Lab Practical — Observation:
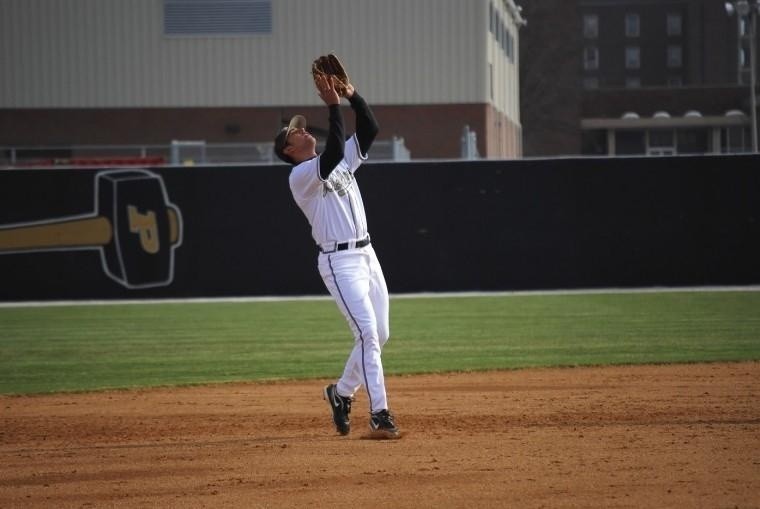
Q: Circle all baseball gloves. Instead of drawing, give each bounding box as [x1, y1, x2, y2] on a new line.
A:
[311, 54, 349, 98]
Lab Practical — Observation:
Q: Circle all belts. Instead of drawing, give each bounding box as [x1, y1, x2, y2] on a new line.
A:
[316, 234, 371, 252]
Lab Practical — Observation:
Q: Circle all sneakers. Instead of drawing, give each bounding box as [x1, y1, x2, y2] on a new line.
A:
[368, 409, 400, 438]
[324, 384, 352, 436]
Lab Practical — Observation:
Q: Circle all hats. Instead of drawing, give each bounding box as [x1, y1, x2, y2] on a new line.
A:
[274, 114, 307, 161]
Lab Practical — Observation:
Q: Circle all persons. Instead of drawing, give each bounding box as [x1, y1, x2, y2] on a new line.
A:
[274, 54, 406, 438]
